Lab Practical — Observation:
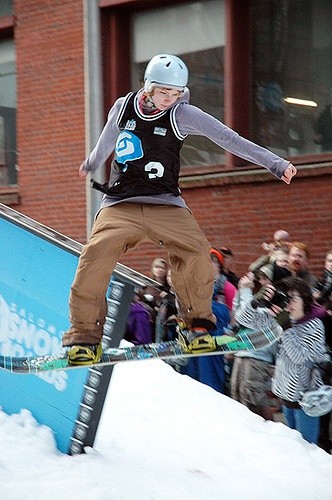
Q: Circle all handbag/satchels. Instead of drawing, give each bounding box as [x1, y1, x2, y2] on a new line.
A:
[297, 364, 332, 417]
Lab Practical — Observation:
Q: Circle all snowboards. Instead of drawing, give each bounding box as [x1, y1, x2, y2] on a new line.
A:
[0, 324, 282, 375]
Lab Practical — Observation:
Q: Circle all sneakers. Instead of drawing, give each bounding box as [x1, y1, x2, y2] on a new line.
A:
[67, 340, 102, 365]
[176, 322, 216, 353]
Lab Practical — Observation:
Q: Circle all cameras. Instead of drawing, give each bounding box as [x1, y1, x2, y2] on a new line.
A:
[267, 287, 290, 308]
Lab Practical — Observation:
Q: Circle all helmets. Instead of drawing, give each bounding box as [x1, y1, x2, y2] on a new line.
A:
[143, 54, 189, 95]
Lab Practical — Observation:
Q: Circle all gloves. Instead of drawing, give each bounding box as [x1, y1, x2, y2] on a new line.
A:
[253, 284, 266, 308]
[274, 311, 292, 331]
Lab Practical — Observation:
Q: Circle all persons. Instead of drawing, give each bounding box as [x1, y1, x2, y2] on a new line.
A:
[311, 251, 332, 316]
[61, 54, 297, 365]
[123, 301, 153, 346]
[160, 269, 178, 341]
[207, 247, 237, 309]
[234, 277, 331, 445]
[284, 242, 318, 289]
[181, 275, 230, 392]
[249, 229, 290, 274]
[139, 258, 169, 312]
[228, 263, 292, 422]
[219, 247, 240, 289]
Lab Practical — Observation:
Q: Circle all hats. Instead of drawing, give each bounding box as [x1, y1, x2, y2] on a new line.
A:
[219, 246, 233, 255]
[210, 246, 225, 265]
